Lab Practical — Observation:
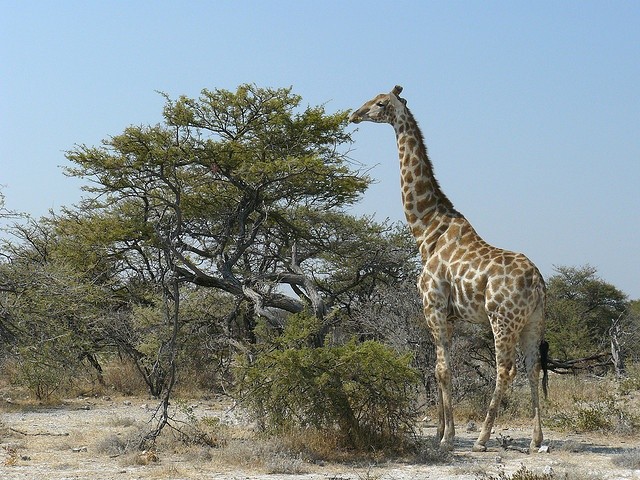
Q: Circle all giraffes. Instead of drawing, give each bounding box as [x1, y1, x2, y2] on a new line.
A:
[350, 85, 549, 453]
